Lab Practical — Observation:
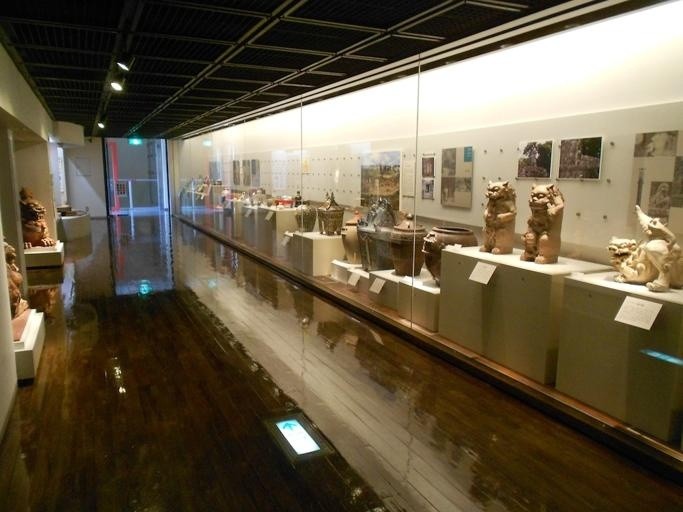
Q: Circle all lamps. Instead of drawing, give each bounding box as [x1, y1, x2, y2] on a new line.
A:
[110, 52, 135, 92]
[97, 114, 109, 129]
